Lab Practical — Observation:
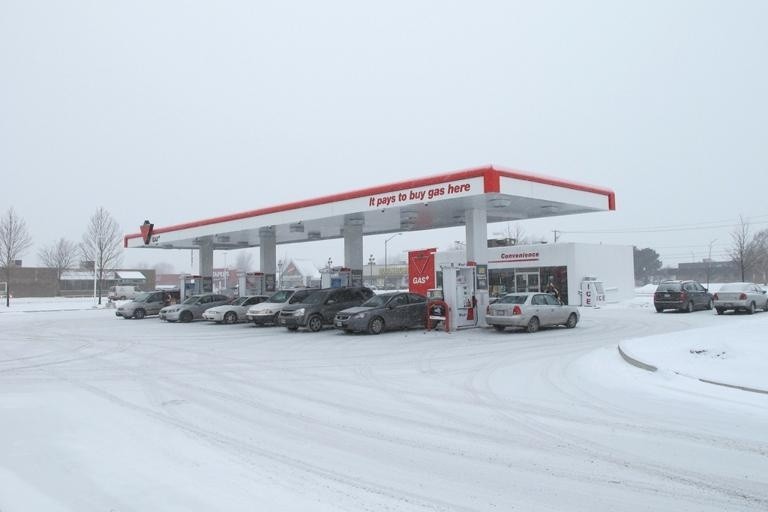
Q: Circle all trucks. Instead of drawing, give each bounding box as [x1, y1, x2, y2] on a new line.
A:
[107, 286, 135, 301]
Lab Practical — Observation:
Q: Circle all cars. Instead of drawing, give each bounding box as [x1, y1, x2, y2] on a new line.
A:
[485, 292, 580, 333]
[654, 280, 768, 314]
